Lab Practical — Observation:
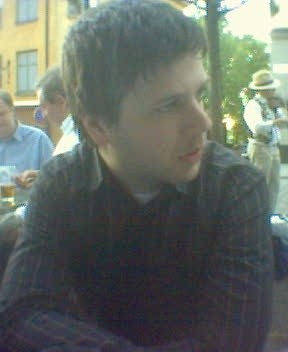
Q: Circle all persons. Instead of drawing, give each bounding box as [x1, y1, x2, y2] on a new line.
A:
[0, 1, 272, 351]
[36, 64, 80, 158]
[244, 69, 287, 228]
[1, 93, 53, 186]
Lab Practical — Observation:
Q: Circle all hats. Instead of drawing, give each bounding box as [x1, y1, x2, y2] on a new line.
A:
[247, 69, 281, 90]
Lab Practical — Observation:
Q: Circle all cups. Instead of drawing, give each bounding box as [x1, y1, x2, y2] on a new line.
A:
[0, 166, 17, 206]
